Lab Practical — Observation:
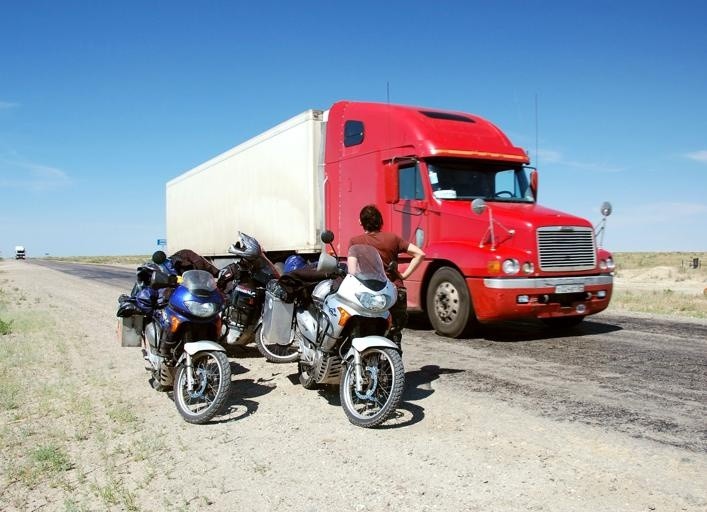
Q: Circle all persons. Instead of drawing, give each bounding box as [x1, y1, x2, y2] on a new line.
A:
[348, 205, 426, 347]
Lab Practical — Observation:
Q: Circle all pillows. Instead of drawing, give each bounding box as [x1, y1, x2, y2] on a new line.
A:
[227, 230, 262, 261]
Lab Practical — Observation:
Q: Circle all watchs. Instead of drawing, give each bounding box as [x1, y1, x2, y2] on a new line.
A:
[117, 249, 234, 420]
[286, 231, 409, 430]
[215, 235, 268, 347]
